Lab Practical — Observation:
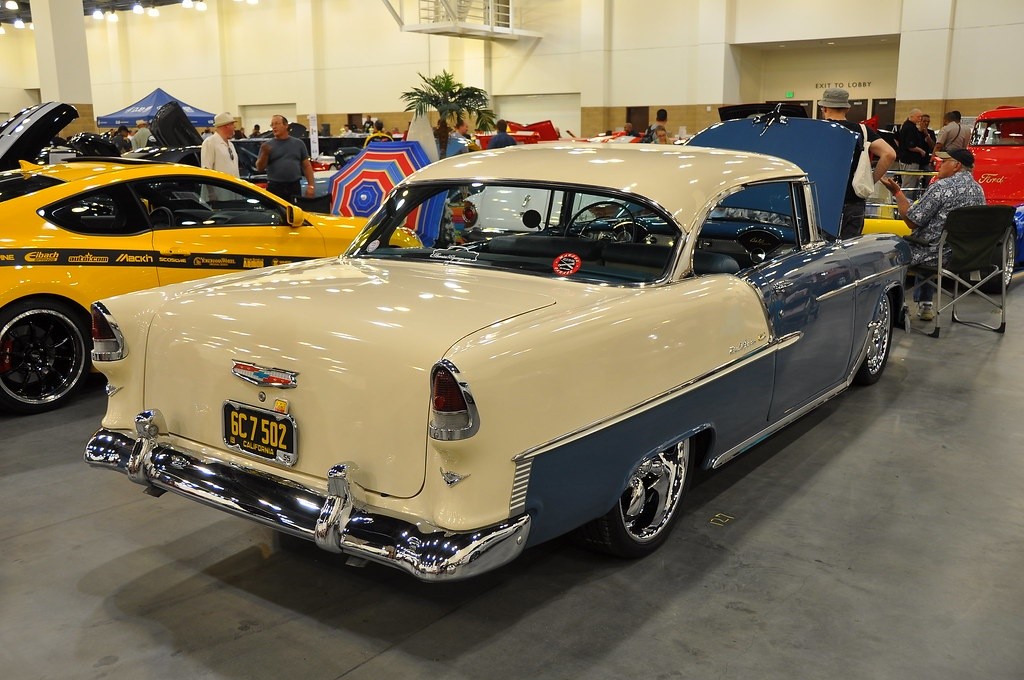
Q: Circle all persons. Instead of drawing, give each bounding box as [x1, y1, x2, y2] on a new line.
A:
[624, 123, 640, 138]
[256, 115, 314, 208]
[102, 120, 153, 153]
[363, 120, 394, 149]
[249, 124, 261, 138]
[880, 148, 986, 320]
[341, 115, 399, 133]
[642, 109, 673, 145]
[818, 87, 896, 239]
[487, 120, 517, 149]
[896, 108, 971, 198]
[604, 130, 612, 136]
[431, 118, 472, 158]
[401, 122, 411, 141]
[199, 112, 246, 210]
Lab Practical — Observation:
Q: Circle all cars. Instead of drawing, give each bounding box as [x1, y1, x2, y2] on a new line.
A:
[0, 158, 427, 414]
[461, 182, 610, 233]
[929, 106, 1024, 209]
[309, 135, 403, 173]
[0, 100, 80, 171]
[109, 103, 341, 213]
[869, 127, 922, 201]
[79, 103, 910, 584]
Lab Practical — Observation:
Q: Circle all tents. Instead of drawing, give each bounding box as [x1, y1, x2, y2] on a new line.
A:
[96, 87, 218, 136]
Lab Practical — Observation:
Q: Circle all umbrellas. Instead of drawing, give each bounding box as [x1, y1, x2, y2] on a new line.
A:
[327, 141, 449, 247]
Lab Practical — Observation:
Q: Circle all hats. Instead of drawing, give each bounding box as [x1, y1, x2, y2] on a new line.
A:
[213, 112, 238, 127]
[818, 88, 851, 108]
[136, 120, 148, 126]
[934, 147, 975, 168]
[119, 125, 130, 133]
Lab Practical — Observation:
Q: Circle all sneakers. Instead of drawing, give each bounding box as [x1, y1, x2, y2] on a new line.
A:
[917, 302, 932, 320]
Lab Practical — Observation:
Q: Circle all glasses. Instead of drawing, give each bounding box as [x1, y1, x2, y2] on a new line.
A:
[228, 148, 235, 161]
[270, 122, 285, 128]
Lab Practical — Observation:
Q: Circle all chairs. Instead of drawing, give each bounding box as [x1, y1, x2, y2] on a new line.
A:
[899, 205, 1017, 339]
[487, 235, 740, 277]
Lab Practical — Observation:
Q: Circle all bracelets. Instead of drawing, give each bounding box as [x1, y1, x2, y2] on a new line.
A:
[308, 185, 314, 189]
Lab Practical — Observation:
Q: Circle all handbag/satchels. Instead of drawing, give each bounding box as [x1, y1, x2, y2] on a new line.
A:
[851, 123, 876, 198]
